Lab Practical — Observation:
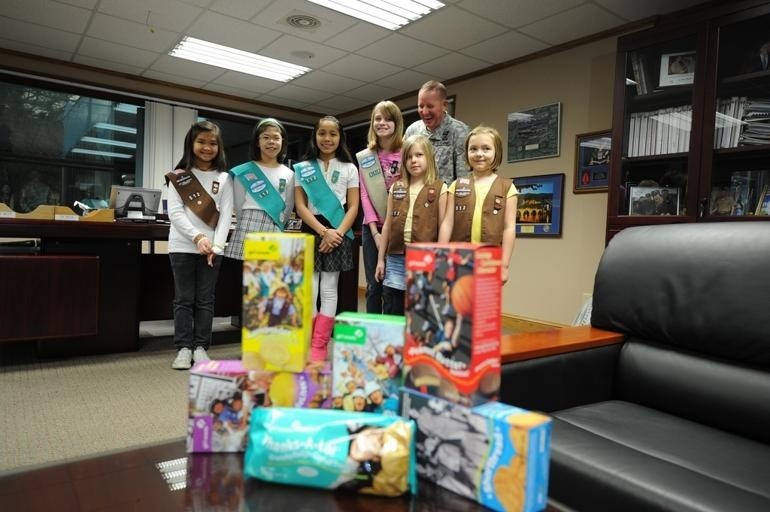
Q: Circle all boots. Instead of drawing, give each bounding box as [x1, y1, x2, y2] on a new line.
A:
[306, 310, 339, 362]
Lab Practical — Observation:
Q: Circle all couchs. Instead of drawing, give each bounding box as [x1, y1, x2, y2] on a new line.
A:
[495, 216, 769, 512]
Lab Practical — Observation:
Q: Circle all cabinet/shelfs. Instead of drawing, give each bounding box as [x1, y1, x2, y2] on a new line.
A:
[603, 2, 770, 250]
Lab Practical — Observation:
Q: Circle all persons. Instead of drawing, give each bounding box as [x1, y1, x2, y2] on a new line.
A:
[437, 126, 521, 286]
[292, 115, 360, 361]
[517, 210, 542, 223]
[401, 80, 471, 187]
[374, 134, 448, 316]
[223, 117, 295, 262]
[752, 43, 770, 72]
[589, 141, 610, 179]
[166, 119, 235, 370]
[354, 100, 405, 312]
[710, 188, 738, 216]
[670, 56, 687, 74]
[632, 189, 676, 216]
[66, 175, 88, 214]
[17, 170, 54, 211]
[0, 183, 16, 212]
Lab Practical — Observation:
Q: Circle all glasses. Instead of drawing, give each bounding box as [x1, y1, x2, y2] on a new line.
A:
[257, 136, 285, 143]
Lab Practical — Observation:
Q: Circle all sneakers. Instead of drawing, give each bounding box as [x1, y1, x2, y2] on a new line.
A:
[193, 346, 212, 366]
[172, 346, 194, 370]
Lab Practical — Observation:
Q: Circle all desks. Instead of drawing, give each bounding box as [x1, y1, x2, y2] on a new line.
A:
[0, 427, 509, 511]
[1, 210, 369, 368]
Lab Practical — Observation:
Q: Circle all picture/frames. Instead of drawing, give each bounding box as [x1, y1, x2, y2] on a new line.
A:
[505, 101, 563, 165]
[571, 128, 610, 195]
[508, 173, 566, 241]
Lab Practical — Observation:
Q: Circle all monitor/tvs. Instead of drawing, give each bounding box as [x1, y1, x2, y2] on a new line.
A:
[109, 185, 162, 215]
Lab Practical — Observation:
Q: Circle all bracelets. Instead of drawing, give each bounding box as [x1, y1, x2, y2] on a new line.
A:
[319, 227, 329, 239]
[372, 232, 379, 238]
